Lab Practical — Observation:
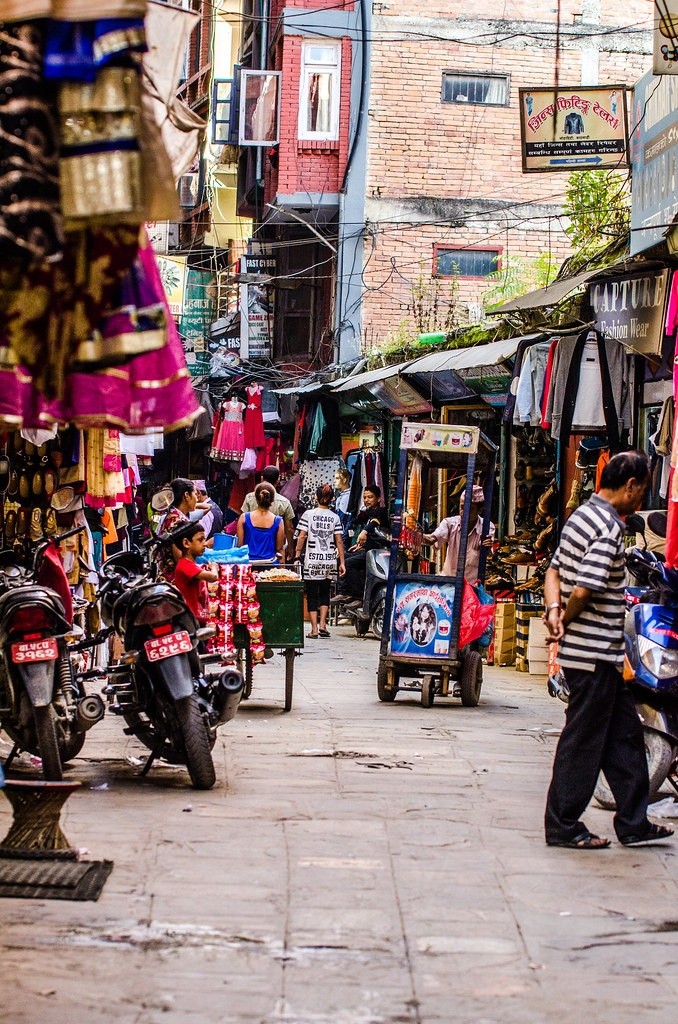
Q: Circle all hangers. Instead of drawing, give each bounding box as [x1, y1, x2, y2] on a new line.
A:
[539, 324, 598, 341]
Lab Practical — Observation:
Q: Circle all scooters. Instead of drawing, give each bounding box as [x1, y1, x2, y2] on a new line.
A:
[346, 519, 391, 639]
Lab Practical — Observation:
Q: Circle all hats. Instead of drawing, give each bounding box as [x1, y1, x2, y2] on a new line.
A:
[459, 485, 485, 503]
[191, 480, 207, 491]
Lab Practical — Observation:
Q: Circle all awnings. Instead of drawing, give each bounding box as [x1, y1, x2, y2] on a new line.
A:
[267, 258, 666, 395]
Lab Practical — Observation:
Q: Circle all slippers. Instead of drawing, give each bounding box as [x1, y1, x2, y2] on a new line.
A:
[318, 628, 331, 638]
[305, 631, 318, 639]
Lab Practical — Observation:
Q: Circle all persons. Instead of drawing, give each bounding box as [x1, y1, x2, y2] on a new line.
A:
[207, 381, 263, 461]
[411, 484, 495, 583]
[171, 523, 218, 656]
[330, 484, 390, 609]
[293, 485, 347, 639]
[236, 482, 284, 570]
[146, 478, 222, 585]
[393, 604, 437, 645]
[542, 451, 675, 847]
[240, 465, 295, 567]
[334, 470, 352, 518]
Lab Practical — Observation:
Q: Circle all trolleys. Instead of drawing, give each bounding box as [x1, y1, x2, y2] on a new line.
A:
[245, 564, 304, 712]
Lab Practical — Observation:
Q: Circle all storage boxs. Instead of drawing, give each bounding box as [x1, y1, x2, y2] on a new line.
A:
[492, 602, 552, 674]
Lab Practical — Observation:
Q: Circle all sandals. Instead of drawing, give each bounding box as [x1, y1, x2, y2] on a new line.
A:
[556, 831, 613, 849]
[623, 823, 674, 845]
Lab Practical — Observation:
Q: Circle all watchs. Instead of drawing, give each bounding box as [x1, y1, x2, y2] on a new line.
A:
[545, 602, 561, 620]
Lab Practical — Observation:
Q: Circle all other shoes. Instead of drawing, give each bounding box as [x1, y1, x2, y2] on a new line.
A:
[330, 594, 352, 604]
[483, 423, 609, 596]
[344, 599, 363, 609]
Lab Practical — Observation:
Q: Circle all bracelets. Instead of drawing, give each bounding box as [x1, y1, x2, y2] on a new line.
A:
[294, 557, 301, 561]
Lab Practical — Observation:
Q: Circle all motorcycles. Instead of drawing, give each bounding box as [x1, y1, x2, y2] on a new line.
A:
[0, 525, 114, 781]
[545, 513, 678, 809]
[93, 508, 246, 791]
